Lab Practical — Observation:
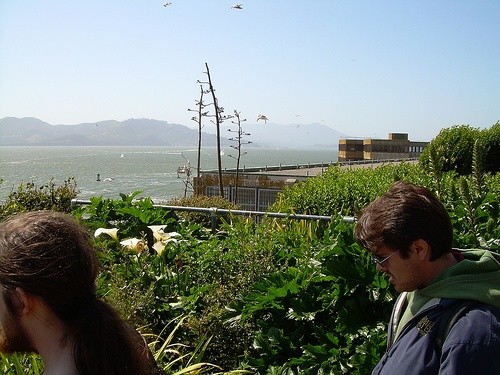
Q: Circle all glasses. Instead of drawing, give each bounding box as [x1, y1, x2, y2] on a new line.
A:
[368, 250, 400, 267]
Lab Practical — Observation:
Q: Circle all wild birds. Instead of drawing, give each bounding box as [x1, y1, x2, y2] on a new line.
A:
[257, 113, 268, 124]
[103, 177, 114, 182]
[229, 3, 243, 9]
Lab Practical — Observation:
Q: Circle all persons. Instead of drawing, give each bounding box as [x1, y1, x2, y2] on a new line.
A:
[351, 180, 500, 375]
[1, 210, 160, 375]
